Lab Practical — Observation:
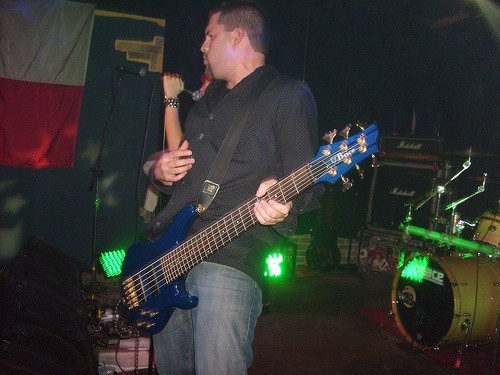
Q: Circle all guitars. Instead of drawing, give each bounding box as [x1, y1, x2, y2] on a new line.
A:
[120, 121, 380, 336]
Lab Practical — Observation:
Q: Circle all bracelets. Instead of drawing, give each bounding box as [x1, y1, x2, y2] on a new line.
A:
[163, 98, 180, 110]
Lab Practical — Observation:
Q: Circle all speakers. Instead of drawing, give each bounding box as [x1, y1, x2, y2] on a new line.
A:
[0, 236, 100, 375]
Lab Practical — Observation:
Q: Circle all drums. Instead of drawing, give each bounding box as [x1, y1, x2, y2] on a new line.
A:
[471, 212, 500, 251]
[392, 251, 500, 354]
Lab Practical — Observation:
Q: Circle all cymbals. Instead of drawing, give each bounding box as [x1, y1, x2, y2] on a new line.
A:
[467, 176, 500, 184]
[448, 151, 500, 157]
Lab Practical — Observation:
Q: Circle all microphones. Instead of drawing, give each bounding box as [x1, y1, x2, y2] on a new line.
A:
[120, 64, 146, 78]
[159, 75, 202, 102]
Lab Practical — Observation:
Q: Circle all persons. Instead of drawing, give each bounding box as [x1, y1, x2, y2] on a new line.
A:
[142, 1, 325, 375]
